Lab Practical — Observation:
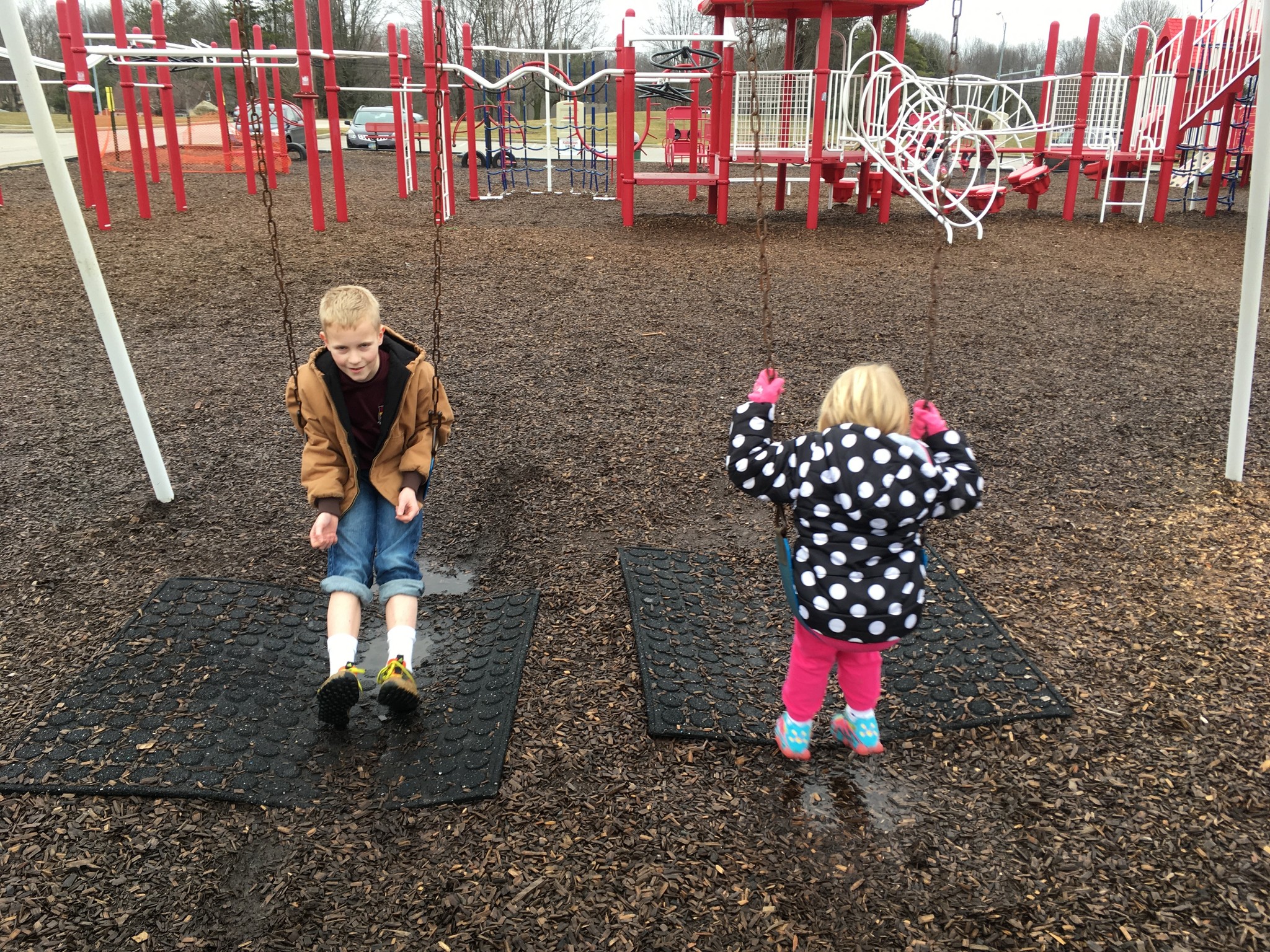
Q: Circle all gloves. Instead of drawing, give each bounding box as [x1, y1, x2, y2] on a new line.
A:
[746, 367, 786, 404]
[909, 399, 949, 441]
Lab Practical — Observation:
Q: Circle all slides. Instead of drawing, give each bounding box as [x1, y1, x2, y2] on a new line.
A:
[1082, 149, 1148, 180]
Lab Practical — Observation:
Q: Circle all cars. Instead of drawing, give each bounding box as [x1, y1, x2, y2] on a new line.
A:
[376, 106, 423, 122]
[234, 102, 258, 119]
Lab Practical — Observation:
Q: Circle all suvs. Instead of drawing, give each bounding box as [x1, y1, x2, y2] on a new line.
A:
[227, 103, 306, 149]
[345, 104, 398, 149]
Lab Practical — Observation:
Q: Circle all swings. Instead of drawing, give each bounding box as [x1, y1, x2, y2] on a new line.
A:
[226, 0, 451, 505]
[737, 0, 964, 643]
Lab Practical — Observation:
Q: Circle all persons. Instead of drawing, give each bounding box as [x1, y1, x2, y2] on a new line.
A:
[285, 285, 453, 728]
[725, 363, 986, 760]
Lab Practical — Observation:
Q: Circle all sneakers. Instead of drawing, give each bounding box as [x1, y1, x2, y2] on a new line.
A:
[315, 661, 366, 726]
[827, 709, 885, 756]
[773, 711, 815, 761]
[376, 655, 421, 714]
